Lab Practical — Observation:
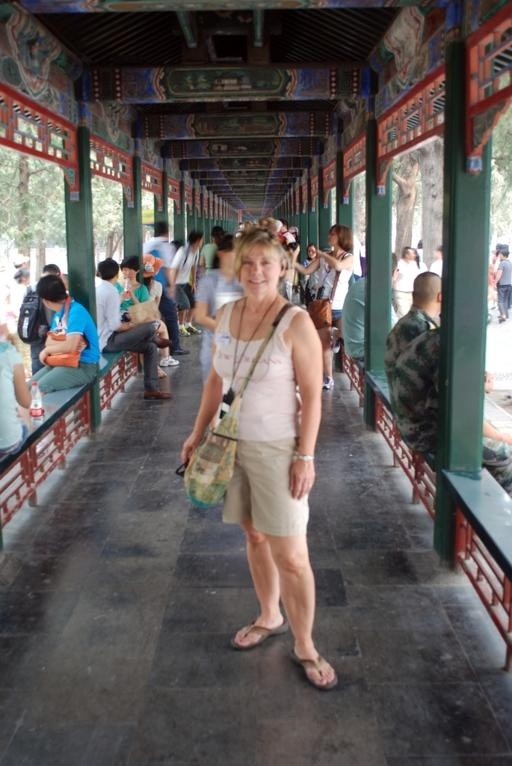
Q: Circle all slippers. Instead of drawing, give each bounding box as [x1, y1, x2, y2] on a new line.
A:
[230, 617, 289, 650]
[287, 646, 338, 691]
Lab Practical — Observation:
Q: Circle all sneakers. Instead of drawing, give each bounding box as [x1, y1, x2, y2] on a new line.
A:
[185, 326, 202, 335]
[178, 327, 190, 337]
[322, 376, 334, 389]
[160, 355, 180, 367]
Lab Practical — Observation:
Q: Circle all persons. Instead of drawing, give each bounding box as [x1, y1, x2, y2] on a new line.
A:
[256, 219, 512, 389]
[385, 271, 511, 499]
[0, 219, 243, 458]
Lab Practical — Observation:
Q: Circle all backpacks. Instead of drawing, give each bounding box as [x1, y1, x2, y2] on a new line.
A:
[17, 291, 51, 345]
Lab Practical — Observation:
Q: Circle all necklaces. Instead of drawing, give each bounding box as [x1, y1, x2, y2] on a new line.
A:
[180, 231, 338, 693]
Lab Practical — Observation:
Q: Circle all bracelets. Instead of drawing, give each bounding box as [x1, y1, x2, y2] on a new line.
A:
[295, 451, 314, 465]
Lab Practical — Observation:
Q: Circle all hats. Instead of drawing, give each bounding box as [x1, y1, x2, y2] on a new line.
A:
[142, 254, 164, 278]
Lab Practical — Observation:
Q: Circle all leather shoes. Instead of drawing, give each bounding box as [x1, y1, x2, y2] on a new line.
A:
[144, 391, 174, 400]
[170, 349, 190, 356]
[153, 336, 173, 349]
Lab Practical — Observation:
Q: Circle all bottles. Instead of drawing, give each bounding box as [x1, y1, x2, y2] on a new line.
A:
[124, 277, 131, 301]
[30, 381, 45, 424]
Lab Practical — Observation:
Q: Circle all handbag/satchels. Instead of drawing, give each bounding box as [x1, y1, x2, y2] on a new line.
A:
[307, 298, 332, 330]
[127, 299, 162, 325]
[183, 413, 240, 510]
[44, 330, 87, 368]
